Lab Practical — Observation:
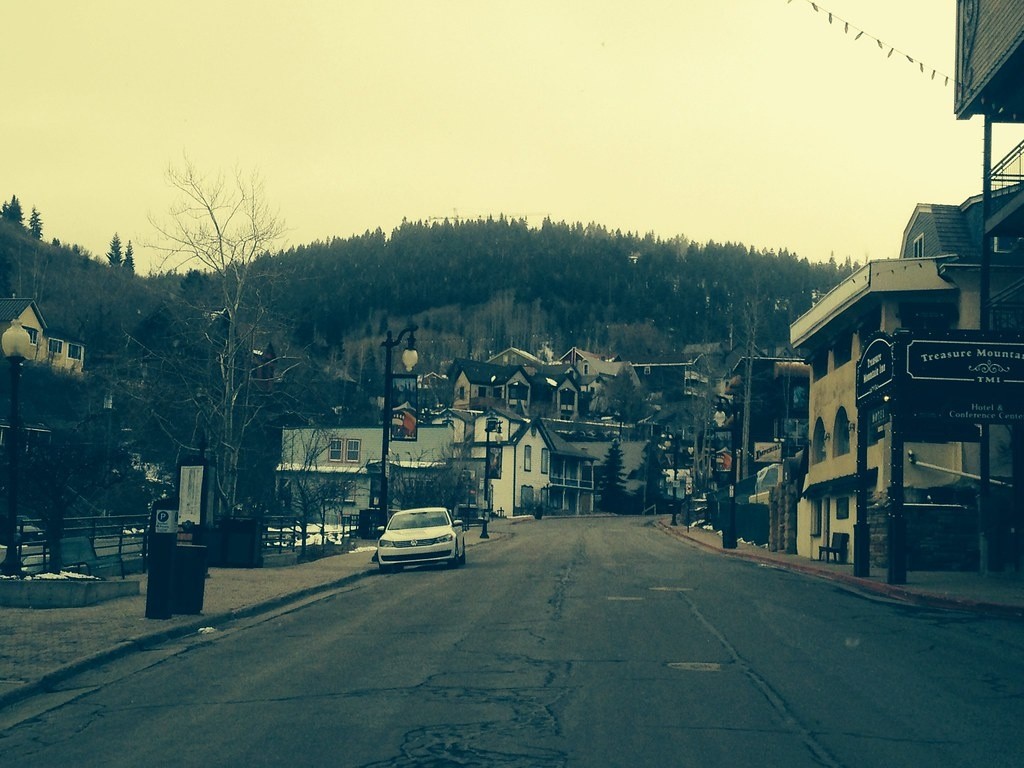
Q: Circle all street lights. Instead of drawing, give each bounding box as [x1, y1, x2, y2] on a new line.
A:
[379, 320, 418, 538]
[1, 319, 34, 578]
[661, 431, 679, 526]
[480, 420, 504, 539]
[714, 395, 738, 550]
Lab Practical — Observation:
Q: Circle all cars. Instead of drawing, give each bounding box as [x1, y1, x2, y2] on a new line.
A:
[377, 507, 466, 573]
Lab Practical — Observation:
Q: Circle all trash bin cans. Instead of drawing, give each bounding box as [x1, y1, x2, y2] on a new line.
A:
[534, 505, 543, 520]
[721, 530, 738, 549]
[172, 544, 208, 615]
[453, 503, 479, 519]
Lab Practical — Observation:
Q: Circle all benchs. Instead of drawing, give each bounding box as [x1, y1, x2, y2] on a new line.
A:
[818, 532, 848, 562]
[458, 507, 483, 530]
[58, 537, 125, 580]
